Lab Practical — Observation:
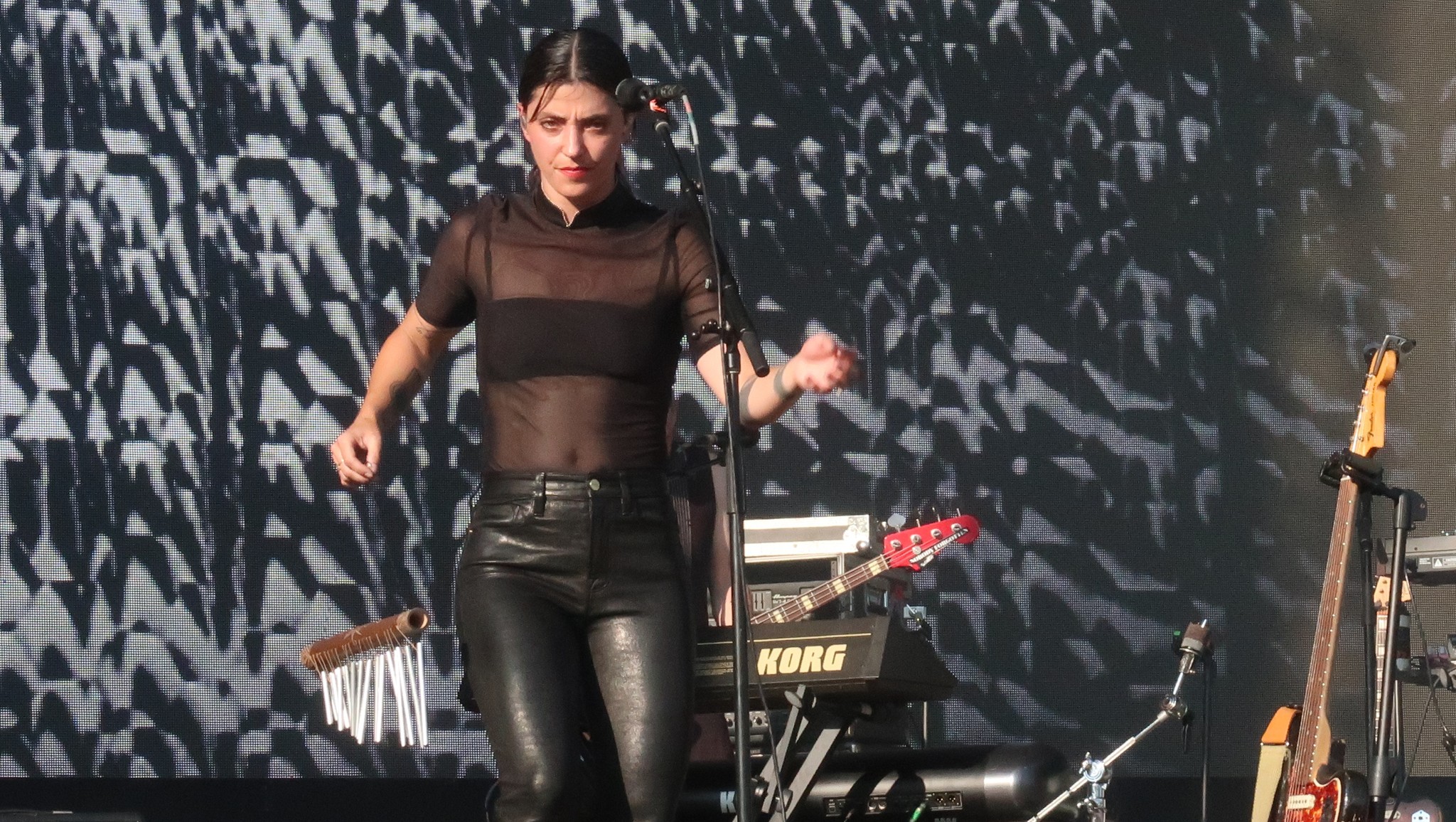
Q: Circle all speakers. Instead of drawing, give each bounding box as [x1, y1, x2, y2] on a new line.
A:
[1374, 539, 1455, 807]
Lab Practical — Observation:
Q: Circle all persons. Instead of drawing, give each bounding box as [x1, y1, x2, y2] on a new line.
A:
[329, 27, 864, 822]
[666, 386, 734, 626]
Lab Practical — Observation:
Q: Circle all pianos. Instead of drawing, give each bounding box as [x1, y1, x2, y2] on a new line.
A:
[456, 618, 956, 820]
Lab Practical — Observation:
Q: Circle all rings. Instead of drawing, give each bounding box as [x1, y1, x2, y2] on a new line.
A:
[337, 458, 344, 466]
[338, 468, 341, 474]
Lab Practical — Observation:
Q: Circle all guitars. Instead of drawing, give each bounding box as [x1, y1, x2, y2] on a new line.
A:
[1372, 574, 1442, 822]
[748, 499, 982, 624]
[1250, 333, 1420, 822]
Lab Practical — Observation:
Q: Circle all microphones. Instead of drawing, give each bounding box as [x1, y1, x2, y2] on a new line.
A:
[614, 79, 687, 113]
